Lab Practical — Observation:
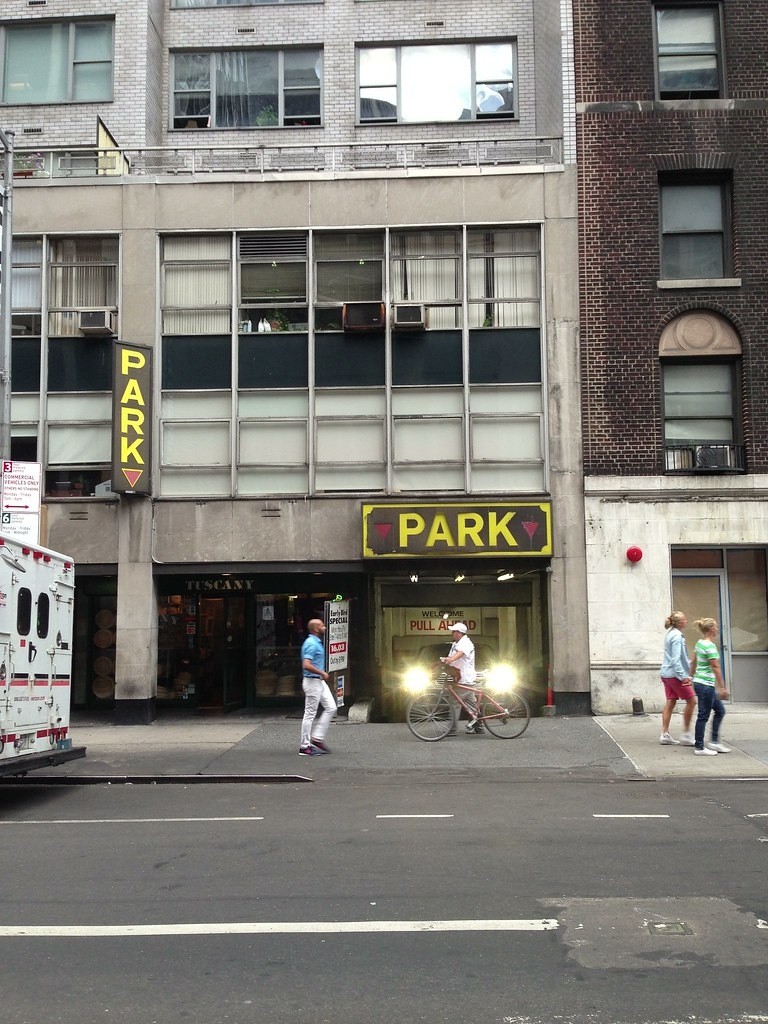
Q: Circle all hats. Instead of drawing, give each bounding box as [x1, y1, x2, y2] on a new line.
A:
[448, 622, 467, 634]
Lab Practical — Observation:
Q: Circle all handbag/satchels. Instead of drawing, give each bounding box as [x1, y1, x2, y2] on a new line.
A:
[437, 666, 461, 685]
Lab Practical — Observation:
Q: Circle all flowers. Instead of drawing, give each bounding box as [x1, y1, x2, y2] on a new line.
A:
[13, 152, 42, 171]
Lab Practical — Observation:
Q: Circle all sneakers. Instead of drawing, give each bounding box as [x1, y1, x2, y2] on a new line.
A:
[680, 734, 696, 745]
[708, 742, 731, 753]
[660, 734, 680, 745]
[310, 740, 330, 753]
[694, 747, 718, 756]
[299, 746, 321, 755]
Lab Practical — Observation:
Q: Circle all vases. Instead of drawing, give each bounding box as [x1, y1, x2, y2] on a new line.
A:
[2, 171, 33, 178]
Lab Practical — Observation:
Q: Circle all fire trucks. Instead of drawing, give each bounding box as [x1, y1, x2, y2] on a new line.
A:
[0, 531, 87, 780]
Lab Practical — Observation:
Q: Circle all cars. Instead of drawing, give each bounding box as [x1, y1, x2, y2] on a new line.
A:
[403, 643, 515, 722]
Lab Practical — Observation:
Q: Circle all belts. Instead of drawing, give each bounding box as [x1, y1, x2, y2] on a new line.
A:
[305, 676, 320, 678]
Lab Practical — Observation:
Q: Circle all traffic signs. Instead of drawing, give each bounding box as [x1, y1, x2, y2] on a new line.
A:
[1, 461, 42, 546]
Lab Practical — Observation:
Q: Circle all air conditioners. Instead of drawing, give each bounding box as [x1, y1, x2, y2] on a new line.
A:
[696, 446, 731, 467]
[78, 310, 112, 334]
[393, 303, 425, 328]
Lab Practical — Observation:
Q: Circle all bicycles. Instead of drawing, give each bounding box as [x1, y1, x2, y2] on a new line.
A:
[406, 658, 531, 742]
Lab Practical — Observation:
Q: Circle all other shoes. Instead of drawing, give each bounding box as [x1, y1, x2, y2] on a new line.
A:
[466, 726, 485, 734]
[441, 730, 456, 736]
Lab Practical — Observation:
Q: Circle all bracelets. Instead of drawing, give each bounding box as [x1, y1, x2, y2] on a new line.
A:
[323, 672, 326, 675]
[688, 676, 693, 680]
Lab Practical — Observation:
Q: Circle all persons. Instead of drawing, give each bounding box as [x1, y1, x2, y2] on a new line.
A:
[659, 610, 697, 745]
[299, 619, 337, 756]
[430, 622, 486, 736]
[689, 618, 732, 756]
